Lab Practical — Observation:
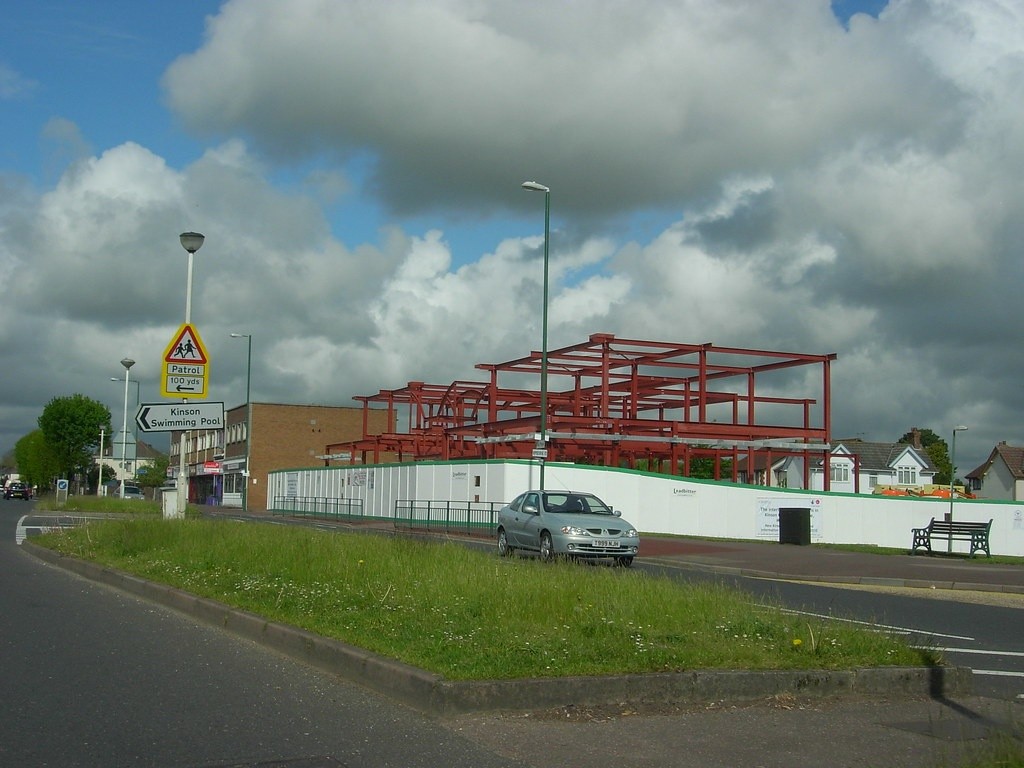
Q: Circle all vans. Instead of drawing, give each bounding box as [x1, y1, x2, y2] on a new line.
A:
[3, 480, 22, 498]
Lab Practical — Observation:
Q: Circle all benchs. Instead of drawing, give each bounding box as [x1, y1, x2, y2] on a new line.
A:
[911, 517, 993, 559]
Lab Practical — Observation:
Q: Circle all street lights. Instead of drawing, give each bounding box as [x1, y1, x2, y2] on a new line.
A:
[120, 358, 137, 500]
[177, 233, 207, 519]
[229, 333, 253, 510]
[110, 377, 140, 486]
[947, 425, 969, 552]
[97, 425, 106, 494]
[521, 181, 551, 488]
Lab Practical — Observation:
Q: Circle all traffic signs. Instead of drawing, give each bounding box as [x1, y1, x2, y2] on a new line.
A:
[162, 362, 208, 399]
[136, 402, 225, 432]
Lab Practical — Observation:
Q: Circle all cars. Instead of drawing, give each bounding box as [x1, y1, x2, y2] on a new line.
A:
[6, 482, 29, 501]
[496, 489, 639, 567]
[112, 485, 145, 500]
